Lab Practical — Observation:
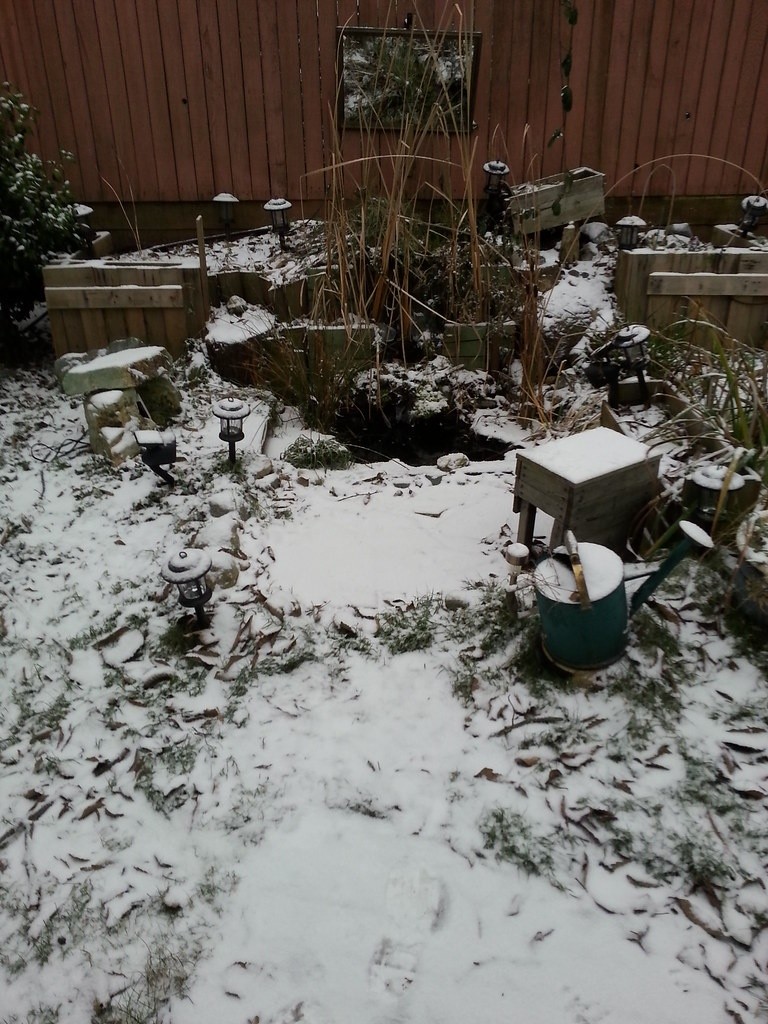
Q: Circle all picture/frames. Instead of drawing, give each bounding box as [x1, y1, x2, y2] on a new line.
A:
[335, 25, 482, 134]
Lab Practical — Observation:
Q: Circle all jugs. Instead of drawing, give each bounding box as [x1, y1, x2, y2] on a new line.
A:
[533, 521, 714, 672]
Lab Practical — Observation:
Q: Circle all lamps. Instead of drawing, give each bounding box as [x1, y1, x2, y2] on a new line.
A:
[616, 215, 646, 249]
[162, 548, 212, 607]
[737, 195, 767, 231]
[692, 464, 744, 521]
[264, 197, 292, 232]
[212, 398, 250, 444]
[73, 202, 95, 230]
[214, 191, 239, 221]
[483, 160, 510, 194]
[616, 323, 652, 368]
